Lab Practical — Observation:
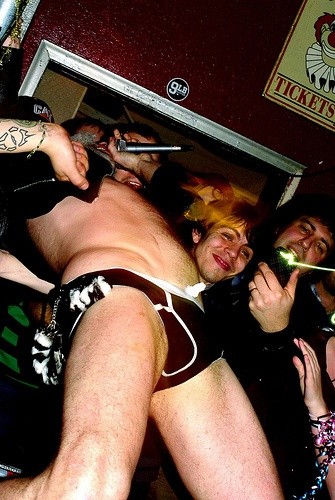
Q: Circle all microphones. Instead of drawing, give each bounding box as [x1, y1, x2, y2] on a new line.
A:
[115, 139, 194, 152]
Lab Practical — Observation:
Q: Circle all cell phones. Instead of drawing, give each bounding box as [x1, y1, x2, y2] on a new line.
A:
[266, 246, 299, 288]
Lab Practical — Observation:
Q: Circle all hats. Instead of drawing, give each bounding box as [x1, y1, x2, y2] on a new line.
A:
[0, 95, 56, 124]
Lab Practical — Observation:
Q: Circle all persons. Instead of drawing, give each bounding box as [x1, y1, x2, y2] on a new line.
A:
[99, 121, 164, 191]
[0, 96, 55, 296]
[1, 116, 108, 480]
[200, 209, 335, 397]
[0, 115, 283, 500]
[0, 119, 89, 190]
[187, 198, 264, 292]
[294, 338, 335, 500]
[310, 270, 335, 315]
